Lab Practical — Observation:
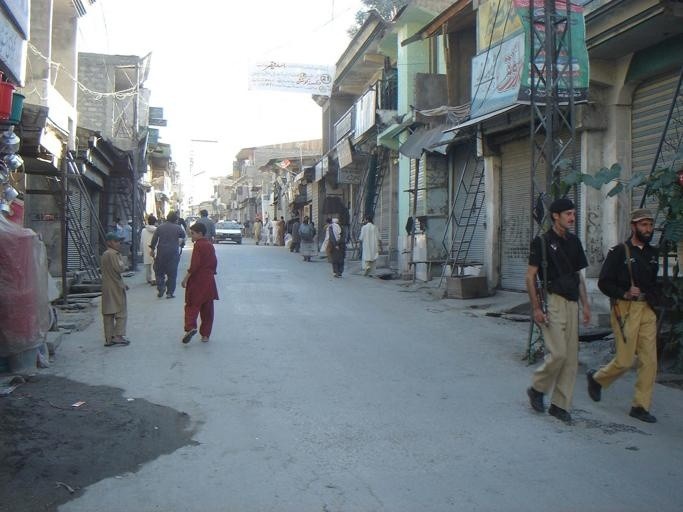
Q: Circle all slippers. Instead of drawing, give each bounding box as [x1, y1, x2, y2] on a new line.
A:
[104, 339, 130, 346]
[182, 328, 197, 343]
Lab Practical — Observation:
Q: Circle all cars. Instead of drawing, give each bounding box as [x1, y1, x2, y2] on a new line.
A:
[212, 220, 242, 244]
[185, 217, 200, 229]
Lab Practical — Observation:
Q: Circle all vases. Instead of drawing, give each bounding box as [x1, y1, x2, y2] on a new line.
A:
[1, 82, 15, 119]
[9, 93, 24, 123]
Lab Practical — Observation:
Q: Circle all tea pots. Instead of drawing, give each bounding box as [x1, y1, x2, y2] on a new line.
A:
[0, 125, 25, 216]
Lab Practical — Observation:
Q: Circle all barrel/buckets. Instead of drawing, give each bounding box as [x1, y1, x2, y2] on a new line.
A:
[0, 71, 26, 126]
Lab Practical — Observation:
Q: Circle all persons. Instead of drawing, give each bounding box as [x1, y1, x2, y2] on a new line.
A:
[180, 222, 219, 344]
[586, 208, 661, 426]
[327, 218, 345, 279]
[358, 215, 383, 279]
[231, 215, 331, 261]
[100, 232, 131, 346]
[523, 198, 592, 422]
[114, 209, 218, 298]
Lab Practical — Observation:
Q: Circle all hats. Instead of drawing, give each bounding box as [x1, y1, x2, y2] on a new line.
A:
[106, 231, 124, 241]
[630, 209, 654, 223]
[550, 198, 575, 212]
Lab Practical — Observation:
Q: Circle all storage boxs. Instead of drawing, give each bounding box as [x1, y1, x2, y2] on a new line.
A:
[447, 275, 487, 299]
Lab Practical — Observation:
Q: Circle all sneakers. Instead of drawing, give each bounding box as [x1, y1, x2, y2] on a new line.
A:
[587, 367, 602, 401]
[527, 386, 545, 411]
[549, 405, 571, 421]
[629, 406, 656, 422]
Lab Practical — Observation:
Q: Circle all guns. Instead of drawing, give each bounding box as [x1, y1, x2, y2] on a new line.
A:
[535, 272, 549, 326]
[609, 297, 626, 342]
